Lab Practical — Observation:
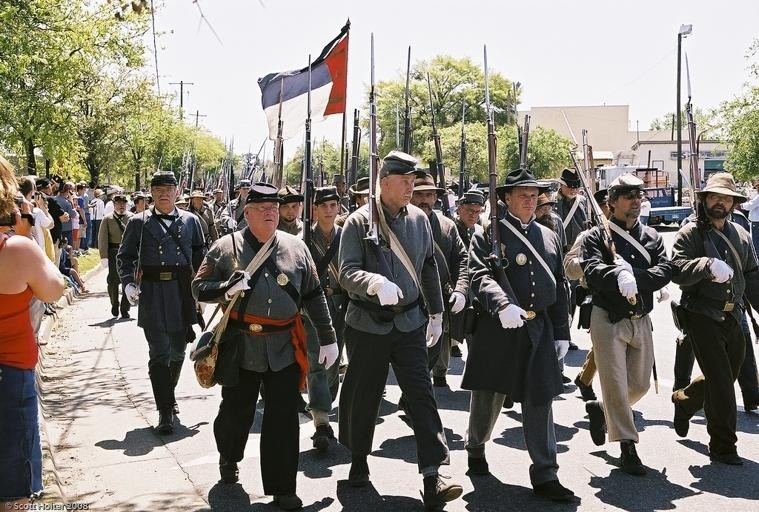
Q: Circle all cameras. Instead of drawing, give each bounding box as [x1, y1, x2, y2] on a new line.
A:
[0, 197, 23, 226]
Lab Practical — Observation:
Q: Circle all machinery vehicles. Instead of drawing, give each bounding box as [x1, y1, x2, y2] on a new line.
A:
[596, 164, 695, 227]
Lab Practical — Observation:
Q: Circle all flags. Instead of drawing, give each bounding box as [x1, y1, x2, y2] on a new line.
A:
[257, 22, 352, 142]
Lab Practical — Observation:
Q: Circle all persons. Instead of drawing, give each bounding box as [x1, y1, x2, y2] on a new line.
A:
[563, 188, 670, 418]
[672, 171, 759, 410]
[0, 155, 65, 511]
[640, 196, 651, 225]
[181, 151, 586, 510]
[14, 169, 152, 320]
[115, 171, 207, 435]
[736, 181, 759, 255]
[670, 172, 759, 465]
[580, 173, 673, 475]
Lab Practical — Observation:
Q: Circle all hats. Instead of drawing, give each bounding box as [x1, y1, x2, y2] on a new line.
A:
[696, 174, 747, 205]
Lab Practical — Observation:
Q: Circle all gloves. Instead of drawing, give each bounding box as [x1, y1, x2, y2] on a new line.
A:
[377, 282, 404, 306]
[124, 283, 141, 305]
[710, 258, 734, 282]
[425, 312, 443, 347]
[498, 304, 528, 330]
[449, 292, 466, 313]
[617, 270, 639, 299]
[227, 269, 252, 295]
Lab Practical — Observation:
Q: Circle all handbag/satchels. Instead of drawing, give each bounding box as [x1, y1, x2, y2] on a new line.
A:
[190, 329, 241, 388]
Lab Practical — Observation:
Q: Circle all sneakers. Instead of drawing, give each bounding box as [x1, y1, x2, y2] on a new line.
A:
[120, 310, 130, 319]
[111, 305, 119, 316]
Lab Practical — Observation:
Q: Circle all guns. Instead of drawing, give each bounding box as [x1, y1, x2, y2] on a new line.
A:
[403, 47, 412, 154]
[272, 78, 282, 189]
[584, 129, 591, 230]
[232, 137, 272, 232]
[157, 137, 234, 210]
[685, 53, 731, 284]
[303, 55, 313, 254]
[561, 109, 637, 306]
[348, 109, 361, 215]
[484, 45, 526, 322]
[458, 100, 465, 198]
[427, 73, 454, 221]
[344, 141, 349, 194]
[518, 115, 530, 170]
[317, 153, 324, 187]
[363, 33, 395, 283]
